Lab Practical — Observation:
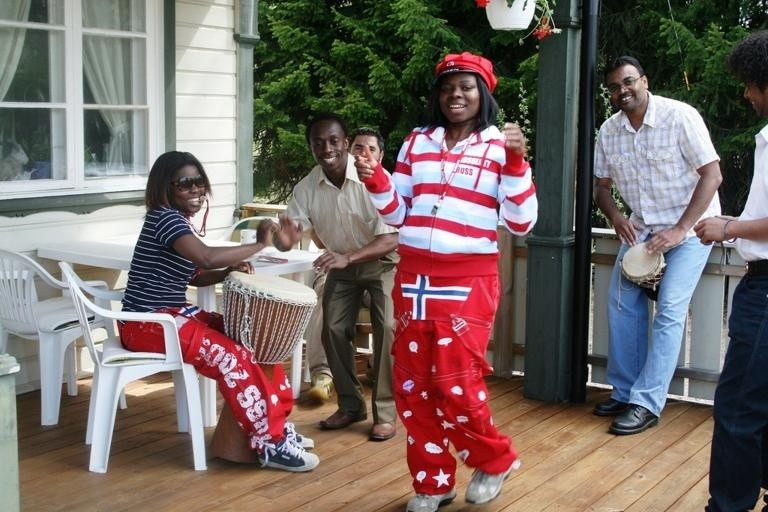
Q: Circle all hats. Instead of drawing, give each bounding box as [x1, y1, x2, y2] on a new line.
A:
[435, 51, 497, 92]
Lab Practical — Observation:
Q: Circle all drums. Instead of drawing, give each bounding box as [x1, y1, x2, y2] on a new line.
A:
[621, 242, 667, 300]
[210, 270, 318, 463]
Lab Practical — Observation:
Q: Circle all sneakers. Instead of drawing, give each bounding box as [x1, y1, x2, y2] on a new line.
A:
[308, 372, 334, 401]
[406, 487, 456, 512]
[283, 420, 315, 449]
[464, 459, 521, 505]
[257, 433, 320, 472]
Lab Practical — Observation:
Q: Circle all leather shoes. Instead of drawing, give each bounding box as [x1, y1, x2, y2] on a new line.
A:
[592, 398, 625, 416]
[319, 410, 367, 429]
[370, 421, 396, 440]
[609, 403, 657, 435]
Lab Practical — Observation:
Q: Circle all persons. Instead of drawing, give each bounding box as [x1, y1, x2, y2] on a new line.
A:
[693, 31, 768, 512]
[115, 149, 319, 474]
[354, 50, 537, 511]
[592, 56, 723, 436]
[306, 127, 393, 403]
[267, 109, 398, 440]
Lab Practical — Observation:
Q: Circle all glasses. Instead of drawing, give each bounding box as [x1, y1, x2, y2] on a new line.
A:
[606, 75, 643, 95]
[172, 173, 206, 192]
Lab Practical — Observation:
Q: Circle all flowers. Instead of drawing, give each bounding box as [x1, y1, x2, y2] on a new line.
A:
[476, 0, 562, 41]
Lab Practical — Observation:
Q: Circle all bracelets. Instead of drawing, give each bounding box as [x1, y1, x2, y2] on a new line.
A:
[724, 219, 737, 244]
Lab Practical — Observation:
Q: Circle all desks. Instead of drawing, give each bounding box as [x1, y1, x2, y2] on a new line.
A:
[0, 356, 21, 512]
[37, 236, 322, 428]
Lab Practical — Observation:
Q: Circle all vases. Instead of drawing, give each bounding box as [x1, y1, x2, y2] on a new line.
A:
[486, 0, 537, 31]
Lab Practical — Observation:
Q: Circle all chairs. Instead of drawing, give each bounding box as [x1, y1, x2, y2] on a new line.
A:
[220, 217, 327, 384]
[58, 260, 207, 474]
[0, 247, 128, 427]
